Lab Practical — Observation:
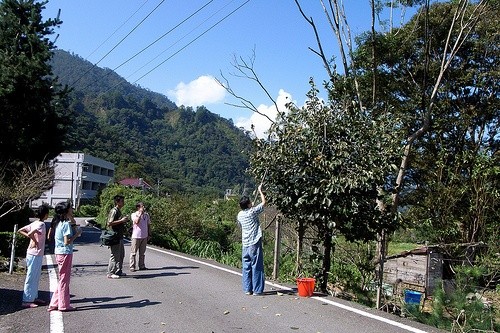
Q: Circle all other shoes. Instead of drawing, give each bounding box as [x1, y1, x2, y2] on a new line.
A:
[107, 273, 120, 279]
[130, 269, 135, 272]
[139, 267, 148, 270]
[22, 302, 38, 308]
[119, 272, 126, 275]
[49, 306, 77, 311]
[245, 291, 263, 296]
[34, 299, 47, 306]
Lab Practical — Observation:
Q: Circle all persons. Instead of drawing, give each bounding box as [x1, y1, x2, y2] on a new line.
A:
[237, 182, 266, 296]
[129, 201, 150, 272]
[42, 201, 83, 311]
[17, 203, 49, 307]
[100, 195, 129, 279]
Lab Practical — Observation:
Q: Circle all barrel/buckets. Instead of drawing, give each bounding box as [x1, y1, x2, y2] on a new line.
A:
[296, 277, 316, 297]
[405, 290, 422, 305]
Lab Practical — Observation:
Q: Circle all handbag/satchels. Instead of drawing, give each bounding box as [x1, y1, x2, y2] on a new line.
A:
[100, 230, 120, 246]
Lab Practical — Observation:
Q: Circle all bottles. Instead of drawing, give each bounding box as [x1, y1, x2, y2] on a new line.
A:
[76, 226, 82, 233]
